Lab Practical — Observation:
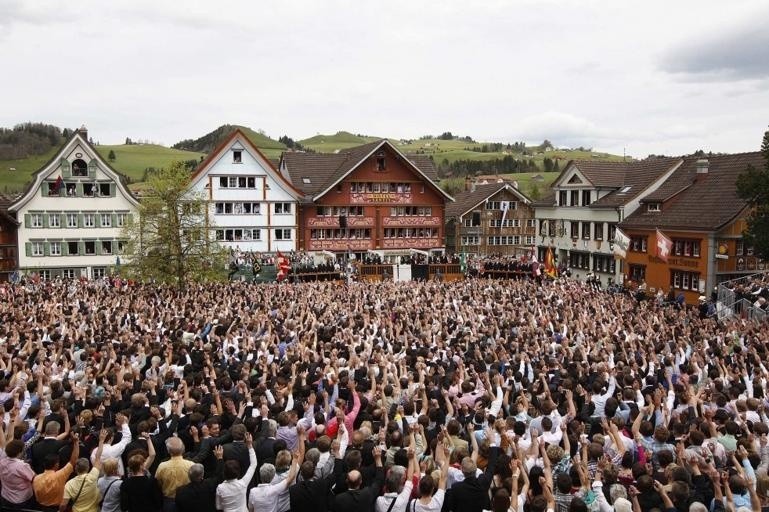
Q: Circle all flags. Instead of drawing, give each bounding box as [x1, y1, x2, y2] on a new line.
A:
[52, 176, 65, 193]
[612, 228, 632, 260]
[656, 229, 674, 260]
